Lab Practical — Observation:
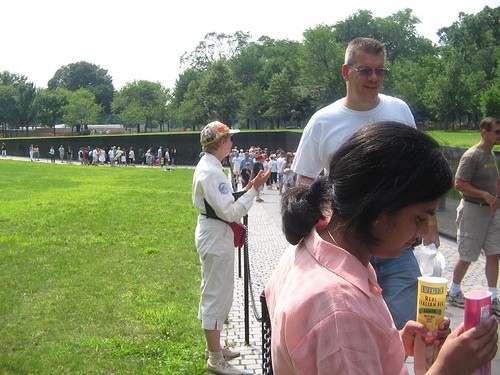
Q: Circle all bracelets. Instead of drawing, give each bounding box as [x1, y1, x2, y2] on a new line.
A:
[429, 220, 438, 225]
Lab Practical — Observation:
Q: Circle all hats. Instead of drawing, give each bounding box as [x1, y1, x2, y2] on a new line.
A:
[256, 155, 265, 159]
[200, 121, 241, 144]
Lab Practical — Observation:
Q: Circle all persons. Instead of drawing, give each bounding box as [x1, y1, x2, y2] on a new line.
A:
[67, 145, 72, 164]
[30, 144, 40, 161]
[108, 146, 134, 166]
[78, 146, 106, 165]
[192, 122, 272, 375]
[59, 145, 64, 163]
[446, 118, 500, 316]
[260, 120, 498, 375]
[49, 145, 55, 163]
[2, 143, 6, 156]
[145, 147, 176, 166]
[230, 146, 297, 202]
[292, 38, 440, 330]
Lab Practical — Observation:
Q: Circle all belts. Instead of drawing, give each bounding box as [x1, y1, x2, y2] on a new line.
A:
[465, 199, 490, 207]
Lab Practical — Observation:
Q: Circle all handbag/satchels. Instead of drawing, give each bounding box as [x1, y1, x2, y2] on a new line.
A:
[414, 238, 445, 277]
[227, 222, 246, 247]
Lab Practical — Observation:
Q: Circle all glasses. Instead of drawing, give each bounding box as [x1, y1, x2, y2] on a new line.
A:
[490, 129, 500, 134]
[230, 135, 233, 142]
[352, 67, 390, 76]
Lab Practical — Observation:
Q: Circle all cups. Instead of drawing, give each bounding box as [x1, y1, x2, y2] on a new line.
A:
[463, 290, 491, 375]
[413, 277, 450, 374]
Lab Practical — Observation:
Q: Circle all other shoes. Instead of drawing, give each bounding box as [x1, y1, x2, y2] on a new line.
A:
[207, 358, 253, 375]
[256, 198, 264, 202]
[489, 297, 500, 316]
[445, 290, 465, 307]
[205, 347, 240, 360]
